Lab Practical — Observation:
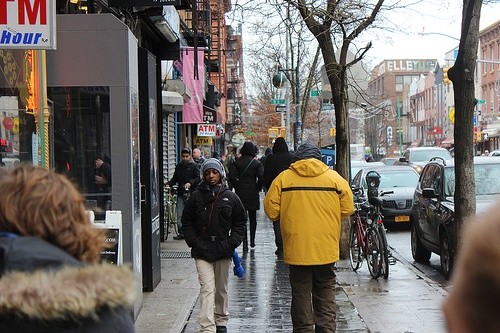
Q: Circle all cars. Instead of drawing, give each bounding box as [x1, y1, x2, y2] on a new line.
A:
[352, 165, 420, 229]
[350, 143, 453, 186]
[487, 149, 500, 157]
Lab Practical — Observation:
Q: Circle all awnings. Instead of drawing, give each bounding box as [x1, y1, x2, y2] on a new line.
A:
[163, 92, 218, 125]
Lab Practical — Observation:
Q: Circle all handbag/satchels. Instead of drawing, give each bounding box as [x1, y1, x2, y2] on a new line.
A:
[234, 178, 239, 188]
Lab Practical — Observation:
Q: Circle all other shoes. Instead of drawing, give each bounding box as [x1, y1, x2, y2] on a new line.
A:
[217, 326, 227, 333]
[173, 235, 184, 240]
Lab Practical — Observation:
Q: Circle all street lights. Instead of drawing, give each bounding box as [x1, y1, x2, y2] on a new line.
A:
[272, 65, 302, 152]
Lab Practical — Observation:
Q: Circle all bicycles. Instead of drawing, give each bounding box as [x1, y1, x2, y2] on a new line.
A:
[160, 184, 186, 241]
[351, 185, 394, 280]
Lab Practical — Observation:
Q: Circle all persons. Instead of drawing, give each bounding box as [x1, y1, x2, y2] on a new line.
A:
[265, 143, 357, 333]
[440, 205, 499, 333]
[364, 154, 372, 162]
[94, 159, 112, 188]
[180, 158, 247, 333]
[170, 137, 299, 260]
[482, 150, 492, 156]
[0, 164, 139, 333]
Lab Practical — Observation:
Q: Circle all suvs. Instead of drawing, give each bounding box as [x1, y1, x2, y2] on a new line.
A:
[408, 155, 500, 280]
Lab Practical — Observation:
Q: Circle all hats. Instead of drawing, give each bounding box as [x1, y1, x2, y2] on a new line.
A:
[202, 162, 221, 176]
